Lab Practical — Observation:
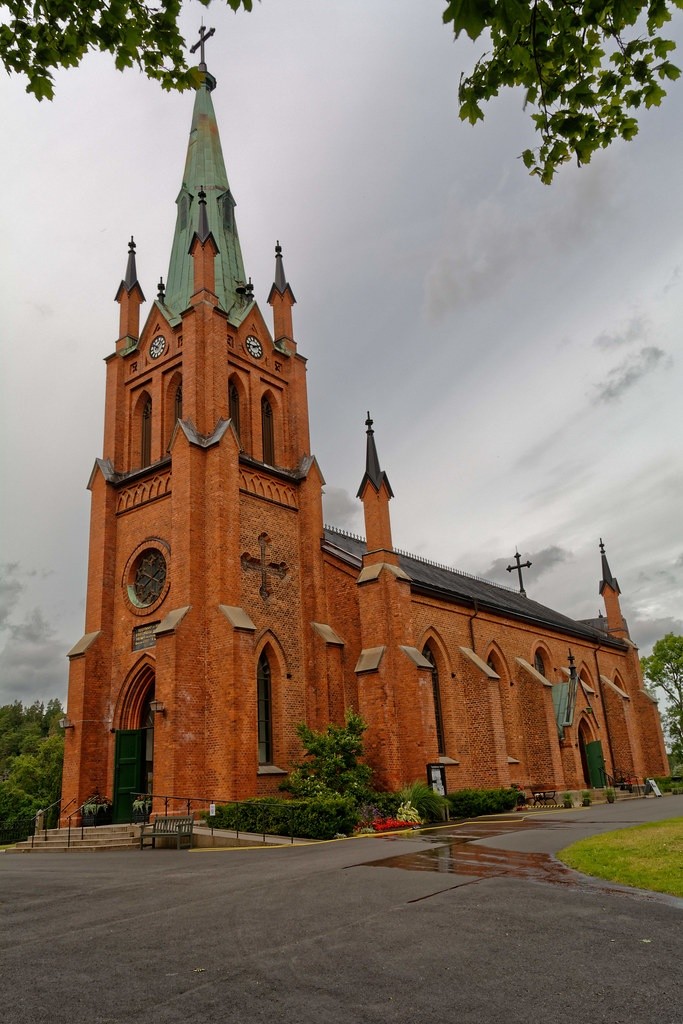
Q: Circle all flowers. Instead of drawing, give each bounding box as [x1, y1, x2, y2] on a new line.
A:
[82, 795, 112, 815]
[133, 793, 152, 814]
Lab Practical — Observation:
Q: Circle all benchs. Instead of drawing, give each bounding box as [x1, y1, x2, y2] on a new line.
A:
[139, 811, 195, 850]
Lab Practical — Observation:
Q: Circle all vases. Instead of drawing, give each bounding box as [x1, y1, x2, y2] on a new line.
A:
[83, 805, 112, 826]
[135, 804, 152, 824]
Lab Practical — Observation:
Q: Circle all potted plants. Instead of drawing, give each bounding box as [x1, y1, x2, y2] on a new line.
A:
[562, 791, 574, 808]
[603, 788, 616, 803]
[581, 791, 593, 806]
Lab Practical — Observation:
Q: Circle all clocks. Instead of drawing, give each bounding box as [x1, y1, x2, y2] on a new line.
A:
[245, 335, 263, 359]
[149, 336, 166, 359]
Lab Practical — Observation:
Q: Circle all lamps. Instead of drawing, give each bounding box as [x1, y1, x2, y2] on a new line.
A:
[150, 699, 164, 712]
[59, 716, 74, 728]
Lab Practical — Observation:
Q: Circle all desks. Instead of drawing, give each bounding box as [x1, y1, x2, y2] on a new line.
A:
[532, 790, 557, 805]
